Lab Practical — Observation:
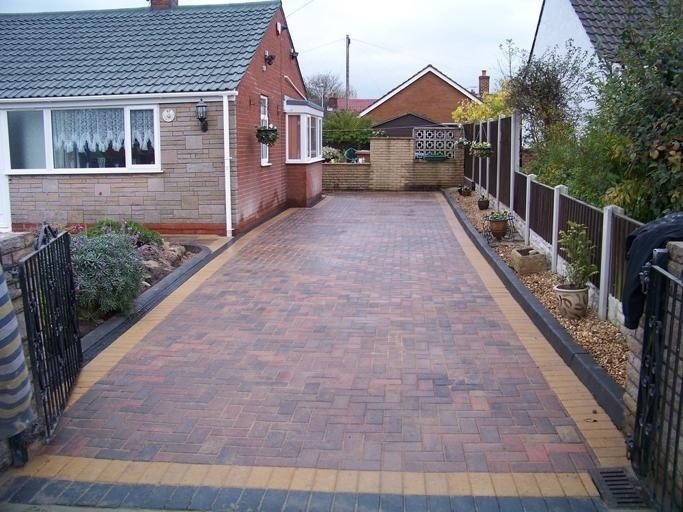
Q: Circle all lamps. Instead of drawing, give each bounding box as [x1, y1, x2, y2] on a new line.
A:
[194, 98, 207, 132]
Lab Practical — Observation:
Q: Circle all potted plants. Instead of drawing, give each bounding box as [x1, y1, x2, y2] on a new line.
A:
[553, 220, 599, 320]
[256, 124, 278, 145]
[488, 211, 508, 241]
